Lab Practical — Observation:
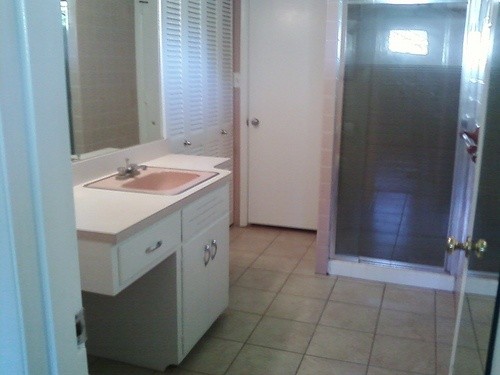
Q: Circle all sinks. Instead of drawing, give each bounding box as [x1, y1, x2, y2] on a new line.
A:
[121, 170, 201, 191]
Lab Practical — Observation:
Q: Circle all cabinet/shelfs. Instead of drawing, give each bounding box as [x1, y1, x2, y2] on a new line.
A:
[85, 184, 231, 372]
[80, 209, 181, 292]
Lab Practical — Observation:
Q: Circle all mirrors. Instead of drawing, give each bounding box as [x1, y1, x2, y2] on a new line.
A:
[60, 1, 164, 162]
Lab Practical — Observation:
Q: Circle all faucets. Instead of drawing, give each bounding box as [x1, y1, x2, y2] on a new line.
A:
[116, 163, 139, 178]
